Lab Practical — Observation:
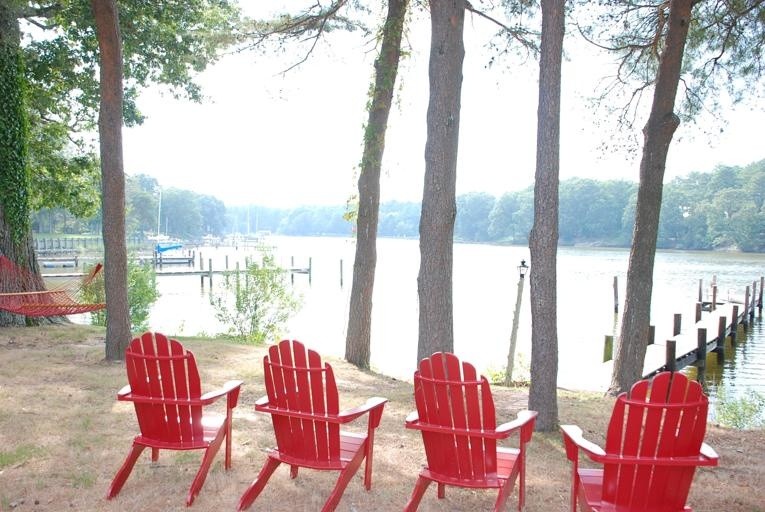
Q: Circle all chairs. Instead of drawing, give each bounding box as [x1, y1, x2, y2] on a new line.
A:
[107, 331, 242, 503]
[234, 342, 392, 509]
[403, 351, 539, 509]
[550, 371, 722, 512]
